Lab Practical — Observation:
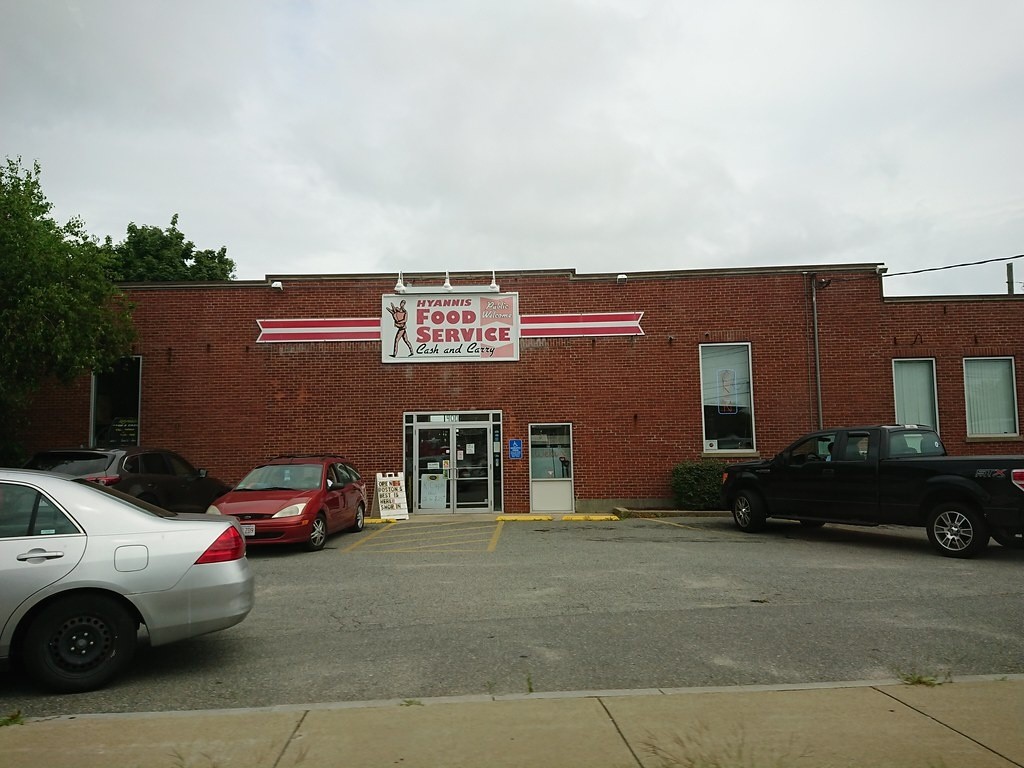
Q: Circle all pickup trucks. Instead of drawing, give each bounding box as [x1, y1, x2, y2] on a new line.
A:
[718, 423, 1024, 559]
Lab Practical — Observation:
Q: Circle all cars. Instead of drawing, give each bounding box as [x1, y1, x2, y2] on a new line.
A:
[20, 443, 231, 515]
[205, 455, 368, 553]
[1, 466, 249, 692]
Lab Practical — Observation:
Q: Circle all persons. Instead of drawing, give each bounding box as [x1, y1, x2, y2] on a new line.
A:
[825, 442, 834, 462]
